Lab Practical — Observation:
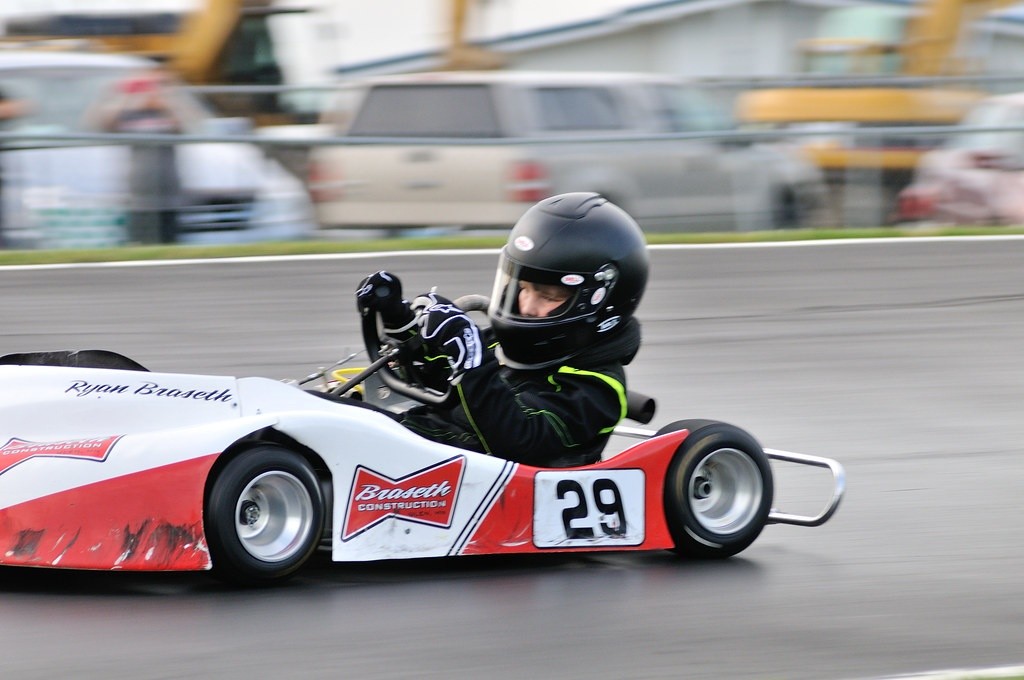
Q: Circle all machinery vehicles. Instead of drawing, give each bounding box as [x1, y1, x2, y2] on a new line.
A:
[736, 0, 1024, 187]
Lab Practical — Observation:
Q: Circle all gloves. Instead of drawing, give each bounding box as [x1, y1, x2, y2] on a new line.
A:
[401, 291, 485, 373]
[353, 270, 400, 317]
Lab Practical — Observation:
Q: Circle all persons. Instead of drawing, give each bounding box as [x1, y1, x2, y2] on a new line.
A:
[0, 92, 29, 119]
[356, 192, 649, 468]
[94, 68, 185, 245]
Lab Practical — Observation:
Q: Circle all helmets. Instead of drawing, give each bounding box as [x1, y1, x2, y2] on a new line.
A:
[488, 191, 651, 372]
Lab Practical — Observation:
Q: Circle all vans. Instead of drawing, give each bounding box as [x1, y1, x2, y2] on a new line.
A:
[0, 52, 313, 250]
[308, 72, 831, 226]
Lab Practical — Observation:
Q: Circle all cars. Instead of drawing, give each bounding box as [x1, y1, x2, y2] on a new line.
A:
[899, 92, 1024, 224]
